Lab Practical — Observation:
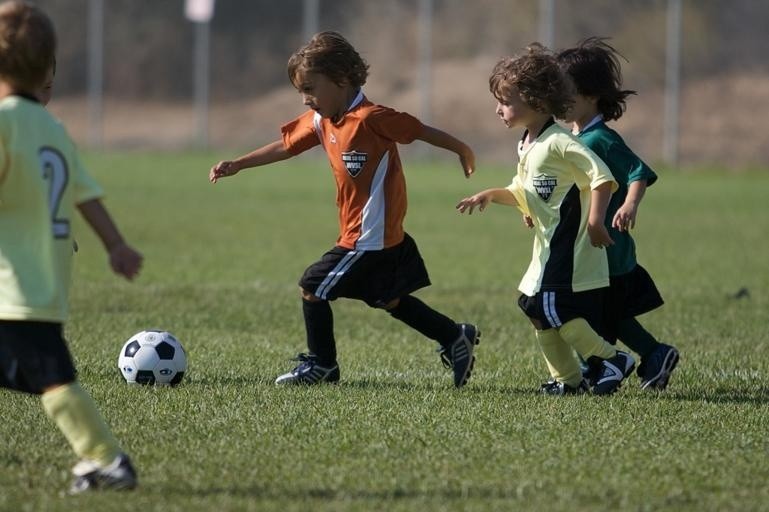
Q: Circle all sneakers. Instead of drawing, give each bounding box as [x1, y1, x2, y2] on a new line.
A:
[58, 453, 136, 495]
[440, 323, 481, 387]
[538, 344, 679, 396]
[275, 353, 340, 387]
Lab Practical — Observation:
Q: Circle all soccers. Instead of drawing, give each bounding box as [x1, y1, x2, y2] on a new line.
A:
[117, 329, 186, 384]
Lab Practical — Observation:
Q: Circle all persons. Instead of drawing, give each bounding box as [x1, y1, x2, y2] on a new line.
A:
[208, 29, 482, 391]
[455, 41, 637, 397]
[0, 0, 144, 496]
[522, 36, 681, 395]
